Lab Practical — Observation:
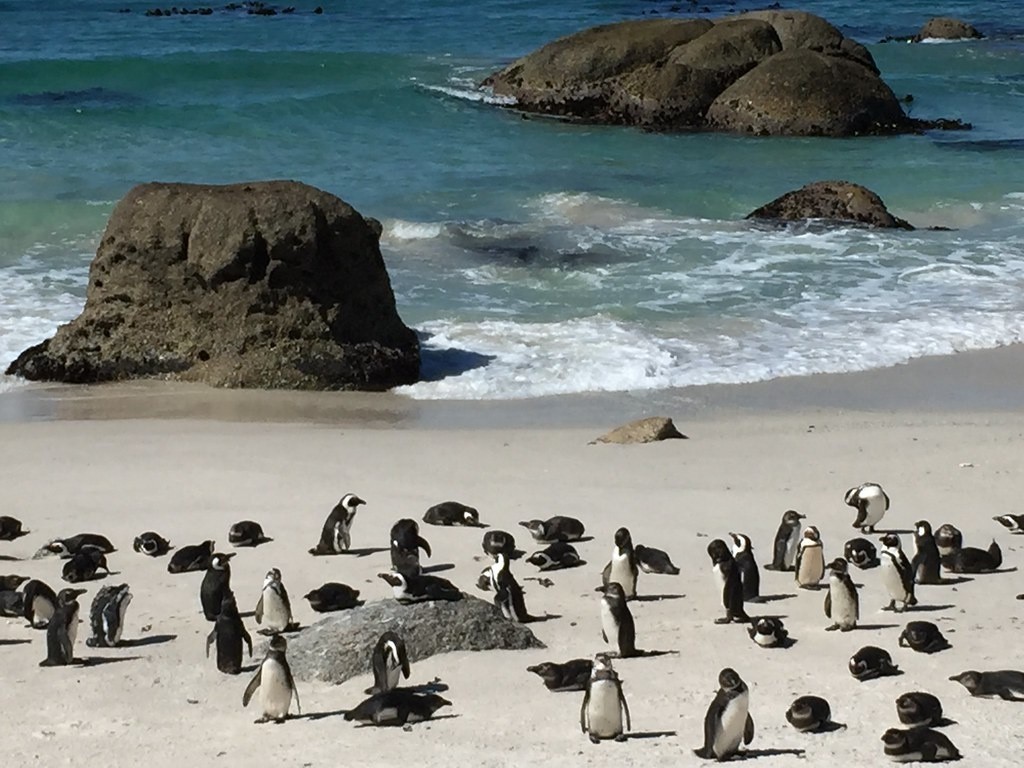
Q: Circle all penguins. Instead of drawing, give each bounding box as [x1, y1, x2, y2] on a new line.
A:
[0, 482, 1024, 764]
